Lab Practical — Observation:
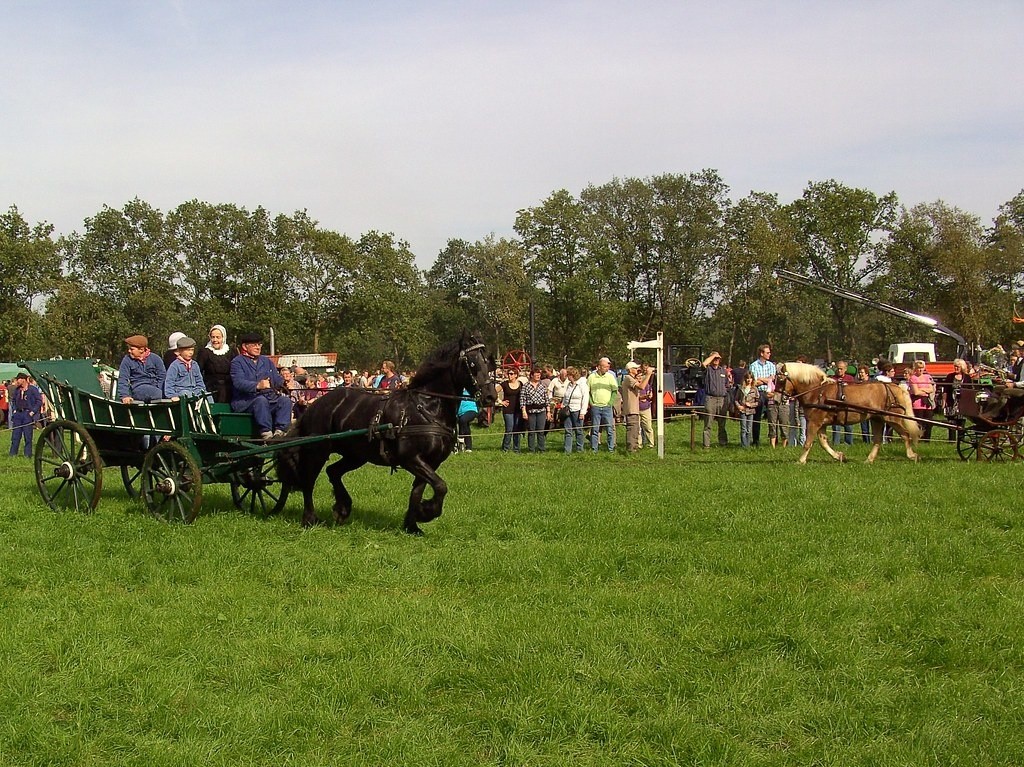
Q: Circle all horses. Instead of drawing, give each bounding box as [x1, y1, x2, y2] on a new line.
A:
[273, 326, 498, 537]
[775, 363, 924, 466]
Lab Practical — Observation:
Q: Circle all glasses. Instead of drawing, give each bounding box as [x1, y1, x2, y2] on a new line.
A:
[507, 373, 516, 375]
[248, 344, 263, 347]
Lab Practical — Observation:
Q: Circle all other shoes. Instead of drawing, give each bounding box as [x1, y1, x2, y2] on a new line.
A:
[457, 437, 464, 443]
[465, 449, 472, 453]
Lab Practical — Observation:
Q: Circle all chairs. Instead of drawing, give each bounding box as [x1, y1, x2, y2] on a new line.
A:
[684, 365, 702, 389]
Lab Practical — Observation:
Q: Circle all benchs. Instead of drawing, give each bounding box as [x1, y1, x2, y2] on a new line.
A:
[121, 394, 266, 435]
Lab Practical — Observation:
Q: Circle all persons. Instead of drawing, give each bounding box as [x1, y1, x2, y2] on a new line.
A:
[942, 358, 973, 443]
[99, 374, 109, 393]
[281, 360, 417, 424]
[701, 345, 807, 448]
[117, 336, 166, 405]
[1005, 340, 1024, 388]
[456, 388, 479, 452]
[164, 337, 214, 403]
[196, 325, 239, 403]
[827, 361, 937, 445]
[162, 332, 187, 399]
[0, 372, 48, 458]
[495, 358, 655, 454]
[230, 333, 292, 439]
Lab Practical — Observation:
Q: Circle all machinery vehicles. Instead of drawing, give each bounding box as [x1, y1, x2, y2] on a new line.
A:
[770, 266, 980, 382]
[651, 344, 710, 423]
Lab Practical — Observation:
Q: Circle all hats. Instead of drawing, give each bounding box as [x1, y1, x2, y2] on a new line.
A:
[168, 331, 187, 350]
[14, 373, 28, 380]
[242, 332, 264, 344]
[599, 357, 612, 364]
[124, 335, 148, 348]
[625, 361, 641, 370]
[177, 337, 196, 349]
[1012, 340, 1024, 349]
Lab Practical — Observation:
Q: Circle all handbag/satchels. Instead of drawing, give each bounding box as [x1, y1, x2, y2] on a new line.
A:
[558, 406, 571, 420]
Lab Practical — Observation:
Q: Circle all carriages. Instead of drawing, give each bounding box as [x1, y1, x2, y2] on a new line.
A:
[16, 325, 499, 539]
[773, 361, 1024, 467]
